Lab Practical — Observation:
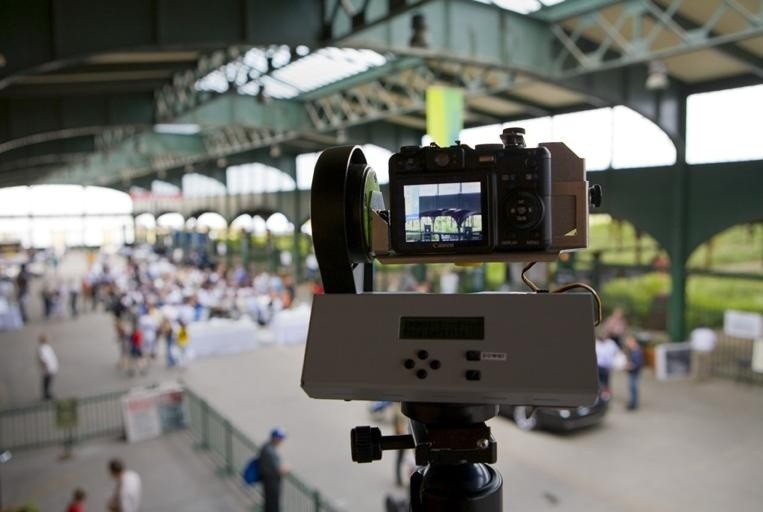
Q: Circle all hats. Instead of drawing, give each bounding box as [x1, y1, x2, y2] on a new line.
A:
[271, 429, 286, 439]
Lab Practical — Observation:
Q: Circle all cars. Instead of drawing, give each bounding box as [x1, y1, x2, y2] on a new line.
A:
[499, 384, 608, 434]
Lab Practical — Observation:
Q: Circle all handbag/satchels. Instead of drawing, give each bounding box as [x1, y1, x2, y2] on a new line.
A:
[243, 459, 261, 484]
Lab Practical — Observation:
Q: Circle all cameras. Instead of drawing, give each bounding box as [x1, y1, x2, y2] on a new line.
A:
[389, 127, 552, 255]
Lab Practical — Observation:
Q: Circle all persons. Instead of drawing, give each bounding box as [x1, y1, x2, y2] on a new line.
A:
[259, 427, 293, 512]
[17, 257, 296, 374]
[66, 457, 143, 512]
[596, 305, 645, 411]
[37, 332, 59, 401]
